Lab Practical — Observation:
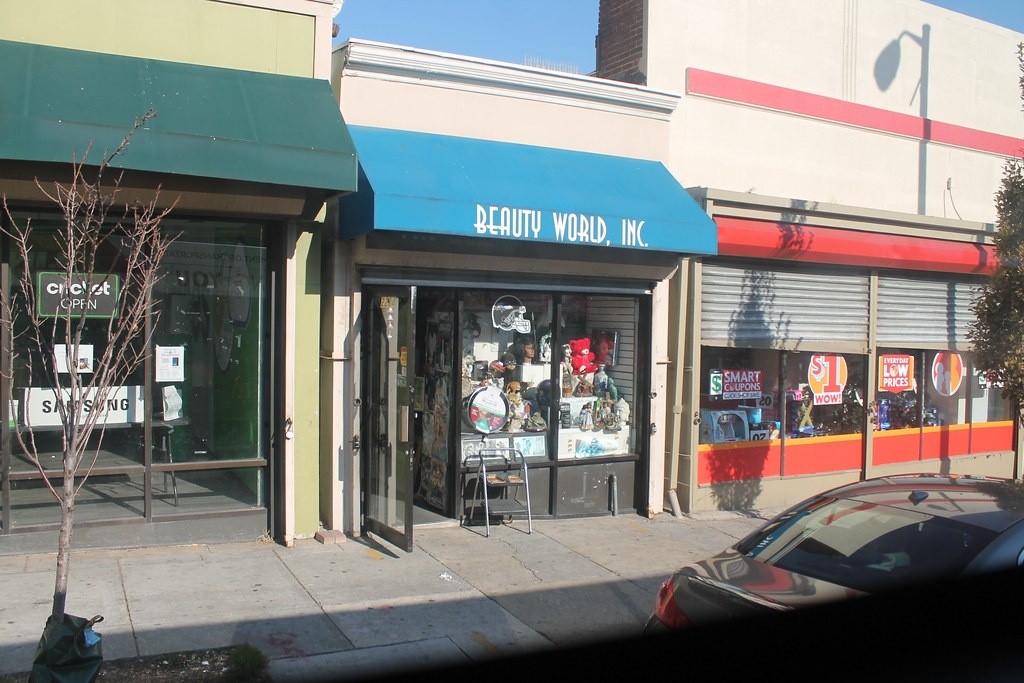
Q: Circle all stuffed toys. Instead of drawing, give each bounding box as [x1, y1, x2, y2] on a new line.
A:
[569, 338, 599, 375]
[562, 343, 574, 374]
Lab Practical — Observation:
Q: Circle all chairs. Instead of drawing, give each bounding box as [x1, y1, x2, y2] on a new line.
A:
[468, 448, 532, 537]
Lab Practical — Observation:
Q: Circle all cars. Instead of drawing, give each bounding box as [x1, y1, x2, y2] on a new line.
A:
[645, 471, 1024, 628]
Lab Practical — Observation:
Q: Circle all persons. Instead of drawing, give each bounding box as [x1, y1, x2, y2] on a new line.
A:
[512, 336, 535, 366]
[501, 350, 517, 371]
